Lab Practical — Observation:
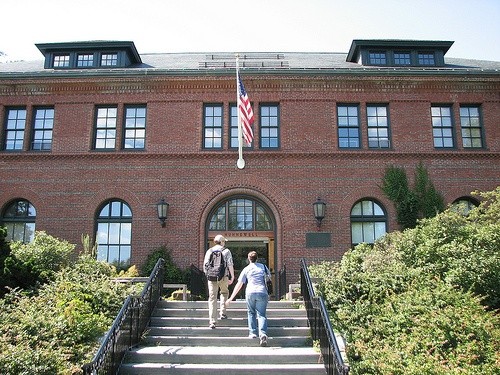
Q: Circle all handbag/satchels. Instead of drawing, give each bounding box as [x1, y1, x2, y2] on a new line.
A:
[264, 265, 275, 294]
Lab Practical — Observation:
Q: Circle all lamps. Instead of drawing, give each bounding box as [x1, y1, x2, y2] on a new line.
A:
[157, 198, 170, 228]
[312, 196, 326, 230]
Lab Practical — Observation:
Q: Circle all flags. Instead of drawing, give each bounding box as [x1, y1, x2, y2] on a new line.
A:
[238, 71, 256, 143]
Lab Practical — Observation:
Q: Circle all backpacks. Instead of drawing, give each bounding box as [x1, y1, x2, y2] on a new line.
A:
[205, 247, 230, 281]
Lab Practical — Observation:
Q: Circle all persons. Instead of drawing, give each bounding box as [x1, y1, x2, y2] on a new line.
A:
[226, 250, 271, 345]
[203, 235, 234, 328]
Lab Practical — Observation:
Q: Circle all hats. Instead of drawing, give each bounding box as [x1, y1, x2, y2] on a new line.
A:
[214, 235, 227, 242]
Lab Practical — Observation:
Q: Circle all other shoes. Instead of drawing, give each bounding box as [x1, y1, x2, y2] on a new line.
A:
[220, 314, 227, 319]
[249, 333, 257, 338]
[260, 333, 267, 347]
[209, 323, 215, 328]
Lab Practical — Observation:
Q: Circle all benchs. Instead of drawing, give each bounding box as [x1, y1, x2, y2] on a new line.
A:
[163, 284, 188, 301]
[289, 283, 300, 299]
